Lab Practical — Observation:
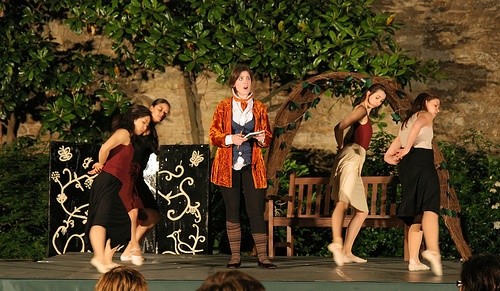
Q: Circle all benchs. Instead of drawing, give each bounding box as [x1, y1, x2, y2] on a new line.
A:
[267, 172, 424, 261]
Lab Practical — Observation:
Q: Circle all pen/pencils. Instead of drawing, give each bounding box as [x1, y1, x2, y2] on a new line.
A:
[237, 130, 243, 150]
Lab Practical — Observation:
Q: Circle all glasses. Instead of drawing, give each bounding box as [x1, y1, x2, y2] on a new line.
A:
[456, 280, 464, 288]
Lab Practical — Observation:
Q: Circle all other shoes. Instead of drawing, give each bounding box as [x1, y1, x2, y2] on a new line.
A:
[258, 261, 277, 269]
[227, 259, 242, 269]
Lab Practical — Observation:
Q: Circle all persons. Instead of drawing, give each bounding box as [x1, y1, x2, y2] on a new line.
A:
[208, 66, 278, 270]
[384, 96, 441, 275]
[95, 264, 147, 291]
[85, 98, 171, 272]
[455, 255, 500, 291]
[195, 270, 266, 291]
[326, 84, 386, 267]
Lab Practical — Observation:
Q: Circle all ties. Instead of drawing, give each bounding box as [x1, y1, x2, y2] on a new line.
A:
[233, 94, 252, 111]
